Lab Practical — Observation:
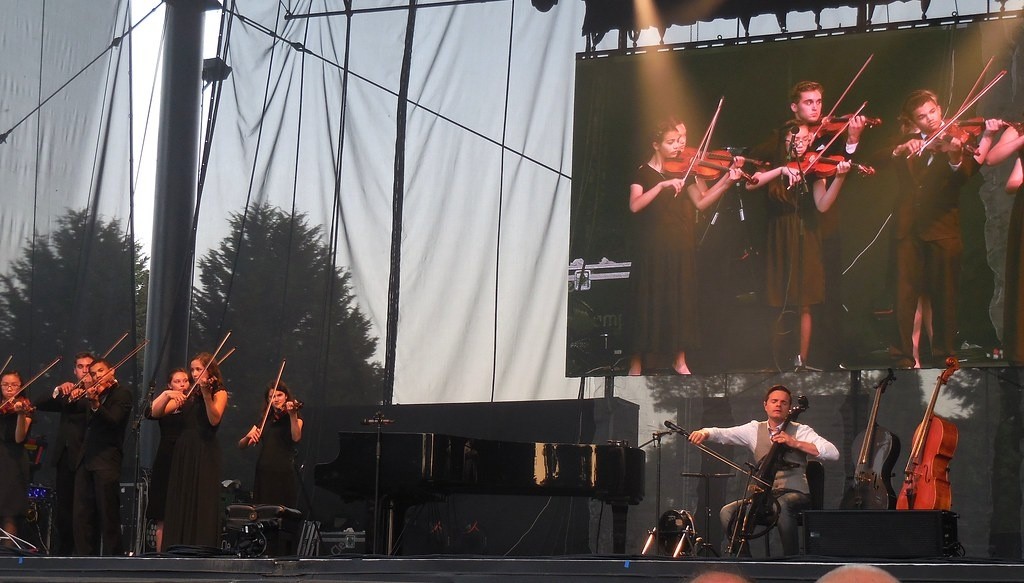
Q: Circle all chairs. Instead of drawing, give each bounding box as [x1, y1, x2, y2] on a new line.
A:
[765, 460, 825, 558]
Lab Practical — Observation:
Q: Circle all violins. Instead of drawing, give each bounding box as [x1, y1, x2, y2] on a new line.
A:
[274, 402, 303, 421]
[808, 114, 883, 139]
[0, 396, 37, 417]
[786, 150, 876, 177]
[662, 146, 773, 185]
[930, 116, 1023, 142]
[183, 376, 218, 397]
[85, 378, 119, 400]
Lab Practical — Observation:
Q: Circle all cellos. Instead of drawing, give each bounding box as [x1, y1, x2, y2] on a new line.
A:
[842, 368, 902, 512]
[896, 356, 964, 512]
[725, 393, 809, 558]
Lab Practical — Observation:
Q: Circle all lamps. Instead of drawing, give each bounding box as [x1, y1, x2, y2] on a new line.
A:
[202, 57, 233, 83]
[531, 0, 558, 13]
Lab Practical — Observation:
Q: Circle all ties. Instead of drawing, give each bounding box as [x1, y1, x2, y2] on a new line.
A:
[919, 135, 932, 170]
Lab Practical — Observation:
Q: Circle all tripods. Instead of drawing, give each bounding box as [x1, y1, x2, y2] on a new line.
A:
[683, 472, 736, 557]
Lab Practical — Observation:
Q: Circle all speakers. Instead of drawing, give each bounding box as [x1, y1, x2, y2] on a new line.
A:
[803, 510, 958, 559]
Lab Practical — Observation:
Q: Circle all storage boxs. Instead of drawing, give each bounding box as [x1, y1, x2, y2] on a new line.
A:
[319, 531, 366, 556]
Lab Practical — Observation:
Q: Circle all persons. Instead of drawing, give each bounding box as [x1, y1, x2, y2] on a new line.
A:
[33, 352, 97, 555]
[692, 564, 898, 583]
[238, 381, 303, 504]
[0, 369, 33, 548]
[629, 81, 1024, 375]
[689, 385, 839, 558]
[151, 352, 227, 553]
[66, 358, 133, 555]
[145, 368, 190, 554]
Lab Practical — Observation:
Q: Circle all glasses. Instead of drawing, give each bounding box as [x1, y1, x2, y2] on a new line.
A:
[786, 139, 809, 146]
[1, 383, 20, 390]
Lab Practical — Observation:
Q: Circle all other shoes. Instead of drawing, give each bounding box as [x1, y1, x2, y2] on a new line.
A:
[671, 364, 691, 375]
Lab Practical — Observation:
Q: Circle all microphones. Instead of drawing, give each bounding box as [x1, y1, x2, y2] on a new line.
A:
[664, 420, 689, 437]
[360, 418, 395, 424]
[787, 126, 799, 163]
